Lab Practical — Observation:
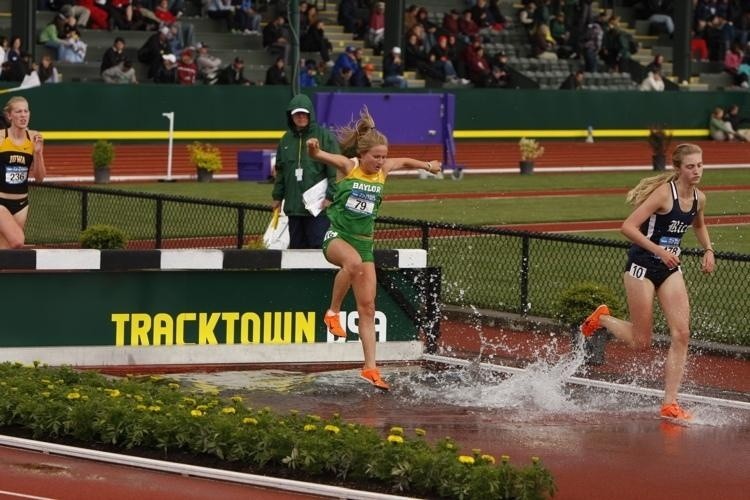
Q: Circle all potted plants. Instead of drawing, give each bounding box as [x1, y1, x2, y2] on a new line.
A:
[512, 134, 546, 177]
[88, 138, 117, 185]
[645, 124, 675, 172]
[546, 280, 625, 368]
[183, 139, 224, 185]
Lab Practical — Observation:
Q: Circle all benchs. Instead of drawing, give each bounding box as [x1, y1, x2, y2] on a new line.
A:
[1, 0, 750, 97]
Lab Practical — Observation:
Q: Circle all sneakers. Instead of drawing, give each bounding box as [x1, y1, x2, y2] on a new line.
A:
[322, 308, 348, 340]
[580, 304, 611, 338]
[358, 365, 389, 392]
[658, 399, 693, 424]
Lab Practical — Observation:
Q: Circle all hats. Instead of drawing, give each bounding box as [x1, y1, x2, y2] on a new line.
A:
[290, 108, 311, 116]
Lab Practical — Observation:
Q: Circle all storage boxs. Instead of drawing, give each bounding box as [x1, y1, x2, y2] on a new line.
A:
[236, 148, 279, 182]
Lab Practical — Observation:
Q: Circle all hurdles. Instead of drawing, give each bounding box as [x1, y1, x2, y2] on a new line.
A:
[0, 249, 442, 367]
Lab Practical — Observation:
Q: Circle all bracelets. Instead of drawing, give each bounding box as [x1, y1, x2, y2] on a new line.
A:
[703, 248, 715, 254]
[427, 161, 433, 171]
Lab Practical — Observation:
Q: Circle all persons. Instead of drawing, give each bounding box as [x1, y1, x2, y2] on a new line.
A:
[0, 97, 46, 251]
[307, 104, 443, 389]
[580, 144, 715, 430]
[709, 105, 734, 141]
[271, 91, 341, 249]
[729, 104, 750, 143]
